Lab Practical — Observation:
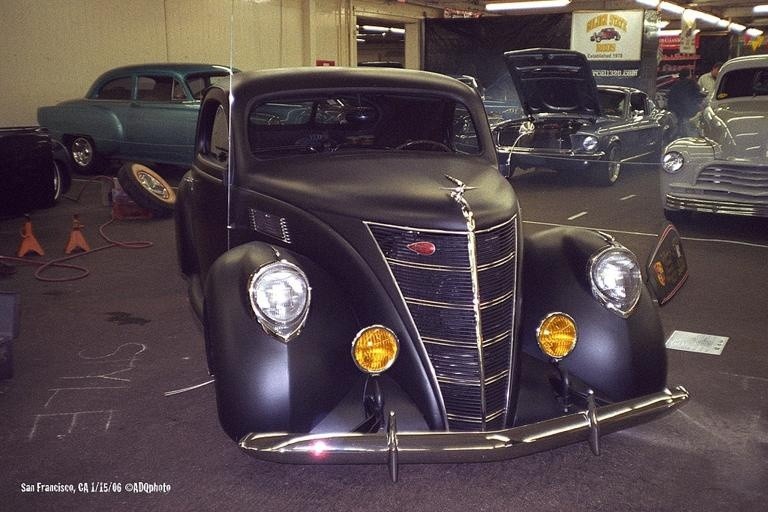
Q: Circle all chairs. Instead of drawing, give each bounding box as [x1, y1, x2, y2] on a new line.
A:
[252, 131, 347, 161]
[100, 82, 172, 100]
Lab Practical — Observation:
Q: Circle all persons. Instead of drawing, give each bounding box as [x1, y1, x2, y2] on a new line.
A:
[697, 63, 723, 103]
[666, 68, 705, 140]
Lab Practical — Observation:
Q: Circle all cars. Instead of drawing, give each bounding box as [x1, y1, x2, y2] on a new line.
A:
[591, 27, 621, 43]
[36, 62, 243, 175]
[660, 55, 768, 221]
[492, 47, 674, 185]
[174, 65, 691, 485]
